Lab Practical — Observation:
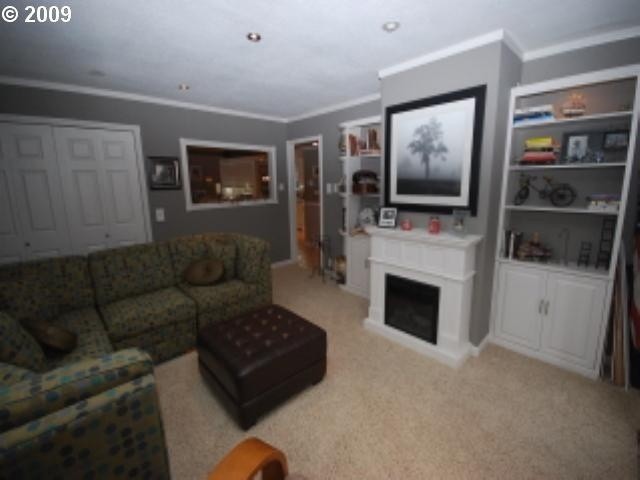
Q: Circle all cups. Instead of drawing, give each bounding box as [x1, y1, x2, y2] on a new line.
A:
[452, 208, 471, 239]
[505, 229, 524, 260]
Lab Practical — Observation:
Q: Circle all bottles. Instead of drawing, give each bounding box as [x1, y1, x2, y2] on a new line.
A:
[429, 216, 440, 234]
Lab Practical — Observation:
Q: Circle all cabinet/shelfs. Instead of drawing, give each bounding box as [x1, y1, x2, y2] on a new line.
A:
[52, 118, 153, 256]
[0, 110, 74, 267]
[338, 114, 383, 299]
[486, 63, 640, 279]
[488, 258, 614, 382]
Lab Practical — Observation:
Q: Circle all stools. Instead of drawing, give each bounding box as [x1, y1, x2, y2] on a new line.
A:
[197, 306, 327, 430]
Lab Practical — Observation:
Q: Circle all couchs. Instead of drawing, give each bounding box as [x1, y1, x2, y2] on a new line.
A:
[0, 258, 170, 480]
[89, 233, 272, 364]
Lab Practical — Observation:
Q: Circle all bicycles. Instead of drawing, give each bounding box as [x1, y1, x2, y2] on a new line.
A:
[514, 175, 576, 207]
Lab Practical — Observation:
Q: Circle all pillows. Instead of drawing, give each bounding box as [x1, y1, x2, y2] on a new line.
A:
[0, 309, 48, 372]
[187, 258, 223, 284]
[207, 238, 236, 278]
[0, 280, 40, 324]
[22, 318, 76, 350]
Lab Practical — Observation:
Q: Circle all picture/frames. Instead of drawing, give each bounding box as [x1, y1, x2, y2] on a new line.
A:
[384, 85, 486, 215]
[147, 157, 182, 191]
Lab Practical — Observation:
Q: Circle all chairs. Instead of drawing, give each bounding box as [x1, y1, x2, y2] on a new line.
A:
[201, 437, 317, 480]
